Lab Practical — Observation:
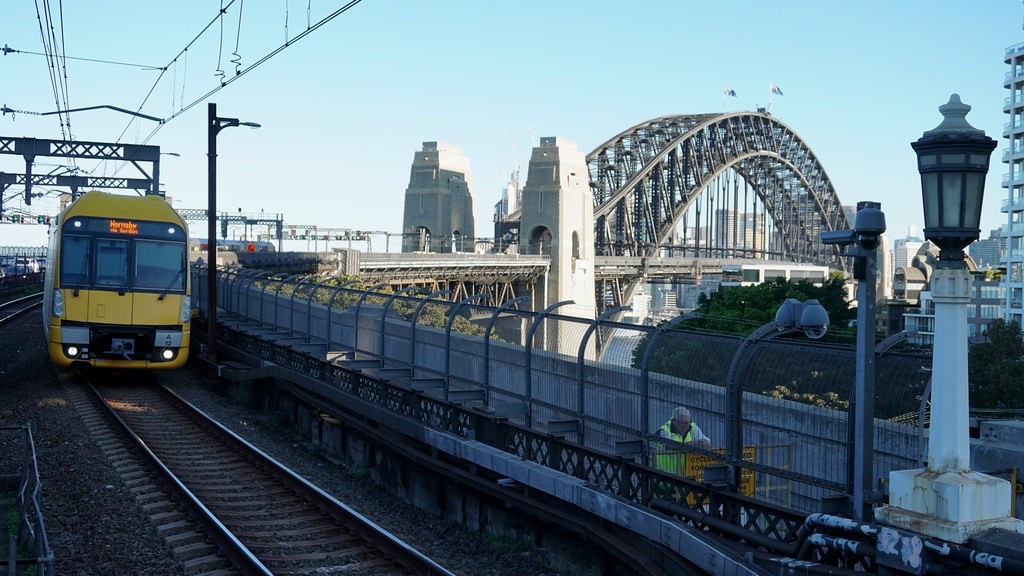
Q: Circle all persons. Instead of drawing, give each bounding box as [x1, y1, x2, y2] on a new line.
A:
[653, 407, 710, 475]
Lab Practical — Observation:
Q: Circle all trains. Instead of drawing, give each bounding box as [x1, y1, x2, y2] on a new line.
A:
[42, 189, 190, 381]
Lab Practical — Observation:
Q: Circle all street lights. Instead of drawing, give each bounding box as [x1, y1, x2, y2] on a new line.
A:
[206, 102, 261, 365]
[873, 92, 1023, 548]
[723, 296, 829, 490]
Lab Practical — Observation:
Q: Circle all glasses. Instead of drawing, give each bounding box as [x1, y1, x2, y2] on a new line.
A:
[674, 419, 691, 424]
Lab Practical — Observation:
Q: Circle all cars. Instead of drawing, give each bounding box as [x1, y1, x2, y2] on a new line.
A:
[11, 257, 39, 273]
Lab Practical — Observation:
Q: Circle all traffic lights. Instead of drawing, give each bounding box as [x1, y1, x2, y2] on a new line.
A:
[37, 214, 46, 224]
[11, 214, 21, 224]
